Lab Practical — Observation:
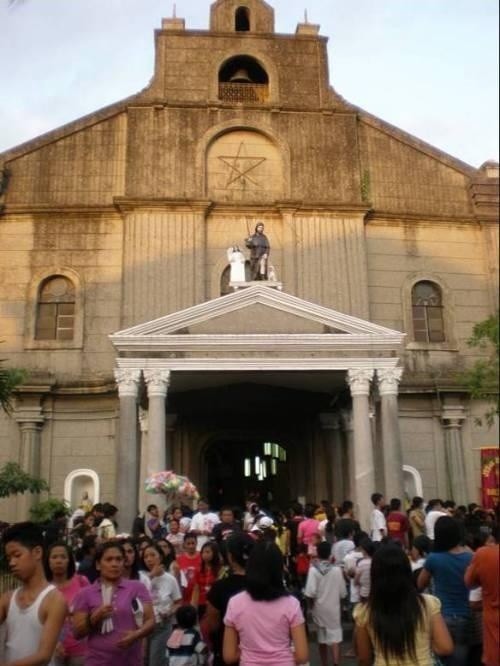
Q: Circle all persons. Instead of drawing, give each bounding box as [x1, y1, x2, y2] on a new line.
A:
[245, 223, 270, 282]
[227, 245, 245, 281]
[0, 491, 500, 666]
